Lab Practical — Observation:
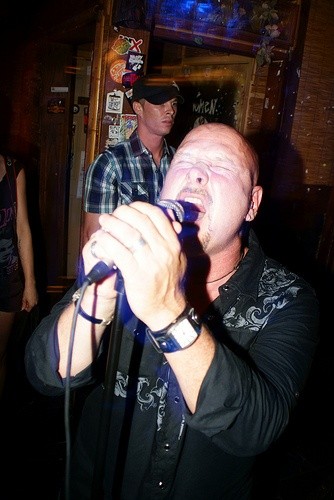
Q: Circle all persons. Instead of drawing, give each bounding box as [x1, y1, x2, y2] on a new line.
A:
[0, 152, 40, 398]
[84, 73, 185, 247]
[25, 123, 320, 500]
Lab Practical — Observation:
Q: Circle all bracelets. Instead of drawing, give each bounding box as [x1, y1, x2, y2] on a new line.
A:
[71, 287, 114, 326]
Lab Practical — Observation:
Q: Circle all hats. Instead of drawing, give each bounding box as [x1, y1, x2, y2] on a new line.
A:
[132, 71, 186, 105]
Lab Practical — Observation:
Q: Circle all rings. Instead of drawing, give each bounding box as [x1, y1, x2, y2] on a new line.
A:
[91, 242, 98, 261]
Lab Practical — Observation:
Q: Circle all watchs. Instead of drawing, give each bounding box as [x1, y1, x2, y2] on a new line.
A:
[144, 303, 202, 354]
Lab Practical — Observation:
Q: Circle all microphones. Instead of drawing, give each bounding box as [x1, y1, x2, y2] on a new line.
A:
[84, 199, 184, 285]
